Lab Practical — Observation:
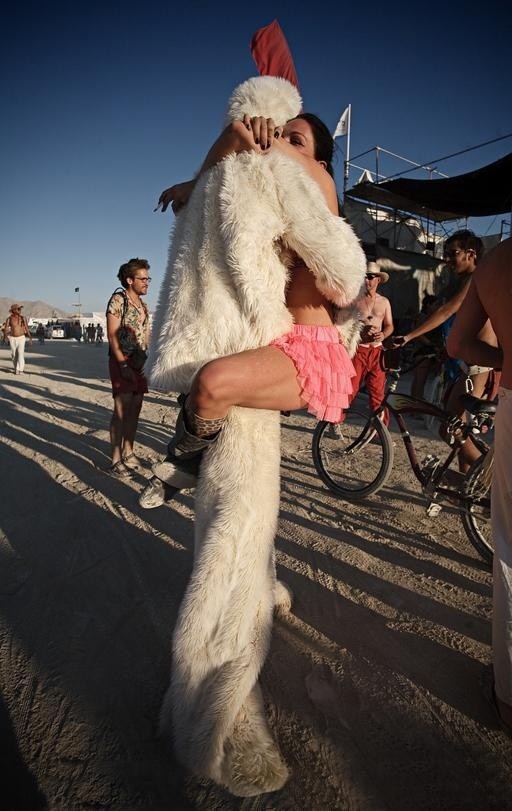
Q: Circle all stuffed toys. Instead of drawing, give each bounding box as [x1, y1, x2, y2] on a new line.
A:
[146, 76, 367, 798]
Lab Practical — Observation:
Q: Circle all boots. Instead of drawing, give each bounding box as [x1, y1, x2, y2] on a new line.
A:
[138, 408, 223, 510]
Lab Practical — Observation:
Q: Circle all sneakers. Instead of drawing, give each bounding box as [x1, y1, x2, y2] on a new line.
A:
[369, 434, 397, 447]
[329, 424, 344, 441]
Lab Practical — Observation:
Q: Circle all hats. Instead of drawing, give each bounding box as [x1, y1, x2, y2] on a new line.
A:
[365, 261, 390, 285]
[9, 304, 23, 311]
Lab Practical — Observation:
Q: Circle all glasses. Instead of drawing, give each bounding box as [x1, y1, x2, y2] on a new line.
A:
[132, 275, 152, 284]
[442, 249, 476, 256]
[367, 275, 376, 279]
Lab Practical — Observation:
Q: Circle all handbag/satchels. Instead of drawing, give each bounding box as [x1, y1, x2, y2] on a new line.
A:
[108, 326, 146, 369]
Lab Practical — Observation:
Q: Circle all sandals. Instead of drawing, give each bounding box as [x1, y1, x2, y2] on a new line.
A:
[111, 451, 141, 478]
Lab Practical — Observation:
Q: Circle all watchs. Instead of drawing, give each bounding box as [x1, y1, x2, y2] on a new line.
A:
[119, 361, 128, 369]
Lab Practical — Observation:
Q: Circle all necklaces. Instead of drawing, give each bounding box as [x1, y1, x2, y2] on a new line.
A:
[129, 294, 142, 309]
[363, 294, 376, 320]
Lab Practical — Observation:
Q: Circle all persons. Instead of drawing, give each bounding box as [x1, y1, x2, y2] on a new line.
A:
[106, 259, 152, 477]
[330, 262, 397, 448]
[1, 320, 104, 346]
[138, 114, 356, 510]
[446, 235, 512, 733]
[387, 230, 502, 507]
[410, 290, 438, 421]
[4, 305, 33, 375]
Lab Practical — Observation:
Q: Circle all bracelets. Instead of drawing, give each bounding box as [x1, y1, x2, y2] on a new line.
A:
[5, 336, 8, 338]
[29, 337, 33, 340]
[403, 336, 408, 343]
[382, 332, 385, 339]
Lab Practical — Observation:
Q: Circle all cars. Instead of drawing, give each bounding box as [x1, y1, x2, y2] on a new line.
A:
[25, 320, 82, 340]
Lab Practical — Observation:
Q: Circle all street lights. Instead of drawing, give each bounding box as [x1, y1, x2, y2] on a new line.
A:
[75, 287, 80, 318]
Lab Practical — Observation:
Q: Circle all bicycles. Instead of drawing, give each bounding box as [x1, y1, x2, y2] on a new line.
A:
[422, 343, 461, 432]
[312, 337, 498, 567]
